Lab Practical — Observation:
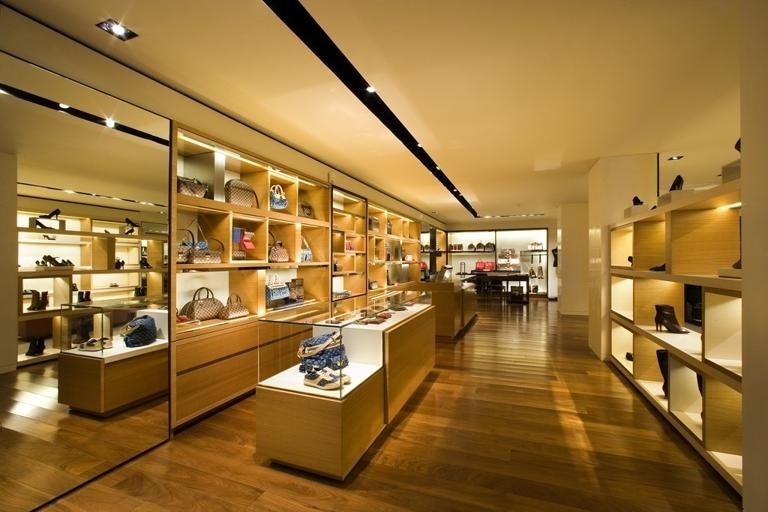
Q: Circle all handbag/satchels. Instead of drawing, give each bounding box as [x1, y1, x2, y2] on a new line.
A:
[224, 178, 260, 208]
[298, 201, 314, 219]
[178, 175, 209, 198]
[334, 260, 343, 271]
[301, 234, 313, 262]
[176, 219, 224, 264]
[468, 242, 495, 251]
[179, 287, 224, 320]
[232, 249, 246, 260]
[369, 216, 381, 232]
[529, 254, 536, 278]
[387, 220, 393, 235]
[370, 280, 379, 290]
[240, 233, 257, 250]
[297, 330, 349, 372]
[269, 184, 288, 209]
[291, 278, 305, 300]
[269, 230, 290, 263]
[537, 254, 544, 279]
[217, 293, 250, 320]
[345, 240, 353, 250]
[266, 280, 290, 308]
[119, 314, 158, 347]
[476, 261, 495, 270]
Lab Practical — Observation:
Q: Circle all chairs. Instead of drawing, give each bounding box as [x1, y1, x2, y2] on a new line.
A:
[469, 267, 530, 303]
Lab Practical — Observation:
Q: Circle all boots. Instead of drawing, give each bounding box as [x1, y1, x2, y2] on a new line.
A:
[655, 348, 669, 398]
[27, 335, 45, 356]
[40, 291, 48, 309]
[78, 291, 86, 305]
[28, 290, 40, 310]
[85, 291, 92, 303]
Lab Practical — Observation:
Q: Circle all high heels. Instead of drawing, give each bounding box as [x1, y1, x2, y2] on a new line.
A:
[655, 303, 689, 334]
[36, 220, 53, 229]
[125, 218, 141, 227]
[39, 208, 60, 218]
[125, 229, 134, 235]
[116, 259, 125, 270]
[36, 254, 75, 267]
[43, 235, 55, 240]
[671, 174, 684, 190]
[141, 256, 152, 268]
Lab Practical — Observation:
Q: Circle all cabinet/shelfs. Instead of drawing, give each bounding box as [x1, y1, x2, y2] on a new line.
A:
[172, 121, 333, 432]
[420, 271, 480, 339]
[446, 231, 496, 282]
[331, 184, 367, 314]
[249, 287, 439, 487]
[53, 295, 169, 418]
[495, 228, 547, 297]
[366, 199, 422, 292]
[17, 218, 170, 366]
[608, 159, 743, 498]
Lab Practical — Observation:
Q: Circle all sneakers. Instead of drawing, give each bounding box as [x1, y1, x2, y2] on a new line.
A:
[650, 262, 666, 271]
[79, 339, 102, 351]
[98, 336, 112, 348]
[304, 366, 351, 389]
[134, 286, 147, 298]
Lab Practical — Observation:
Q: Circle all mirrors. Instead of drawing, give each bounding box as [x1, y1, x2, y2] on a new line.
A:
[0, 48, 174, 511]
[419, 219, 446, 284]
